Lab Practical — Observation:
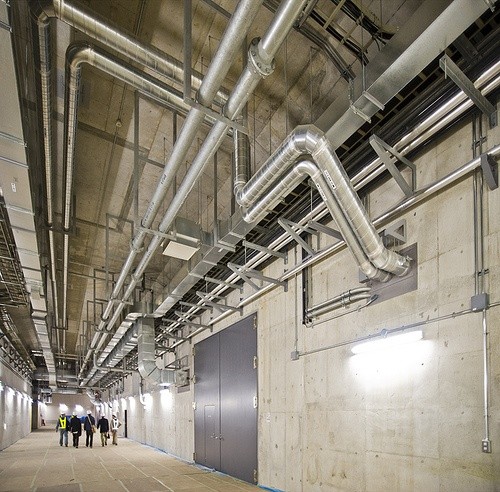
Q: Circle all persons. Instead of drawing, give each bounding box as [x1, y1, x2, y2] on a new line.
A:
[56, 411, 70, 447]
[97, 413, 109, 447]
[110, 412, 121, 446]
[84, 410, 95, 448]
[70, 411, 82, 448]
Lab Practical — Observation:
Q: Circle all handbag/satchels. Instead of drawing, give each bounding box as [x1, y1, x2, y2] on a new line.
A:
[107, 434, 110, 438]
[91, 425, 96, 434]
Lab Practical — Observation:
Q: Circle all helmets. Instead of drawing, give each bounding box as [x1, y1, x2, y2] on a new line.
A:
[61, 412, 65, 414]
[87, 410, 92, 414]
[73, 411, 78, 415]
